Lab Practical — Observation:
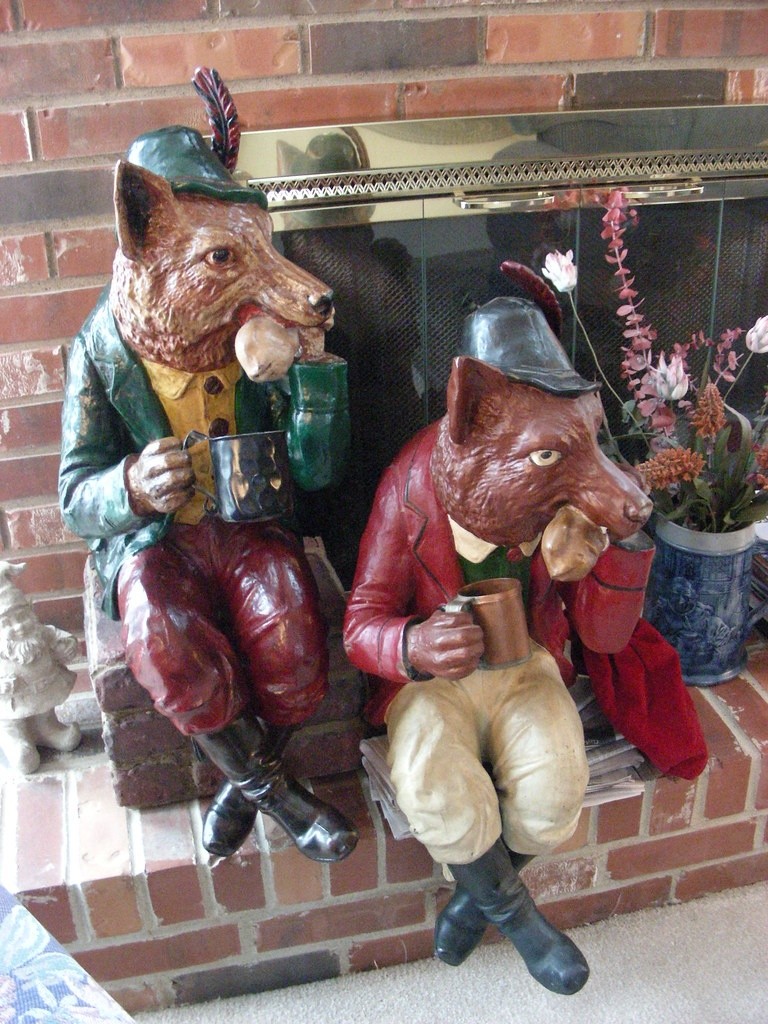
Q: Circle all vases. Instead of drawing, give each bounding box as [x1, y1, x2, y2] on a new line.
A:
[645, 509, 755, 686]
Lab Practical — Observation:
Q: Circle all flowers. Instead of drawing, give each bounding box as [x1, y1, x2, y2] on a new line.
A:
[540, 186, 768, 535]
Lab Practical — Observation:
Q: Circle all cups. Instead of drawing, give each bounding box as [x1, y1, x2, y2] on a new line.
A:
[444, 577, 533, 670]
[181, 429, 296, 525]
